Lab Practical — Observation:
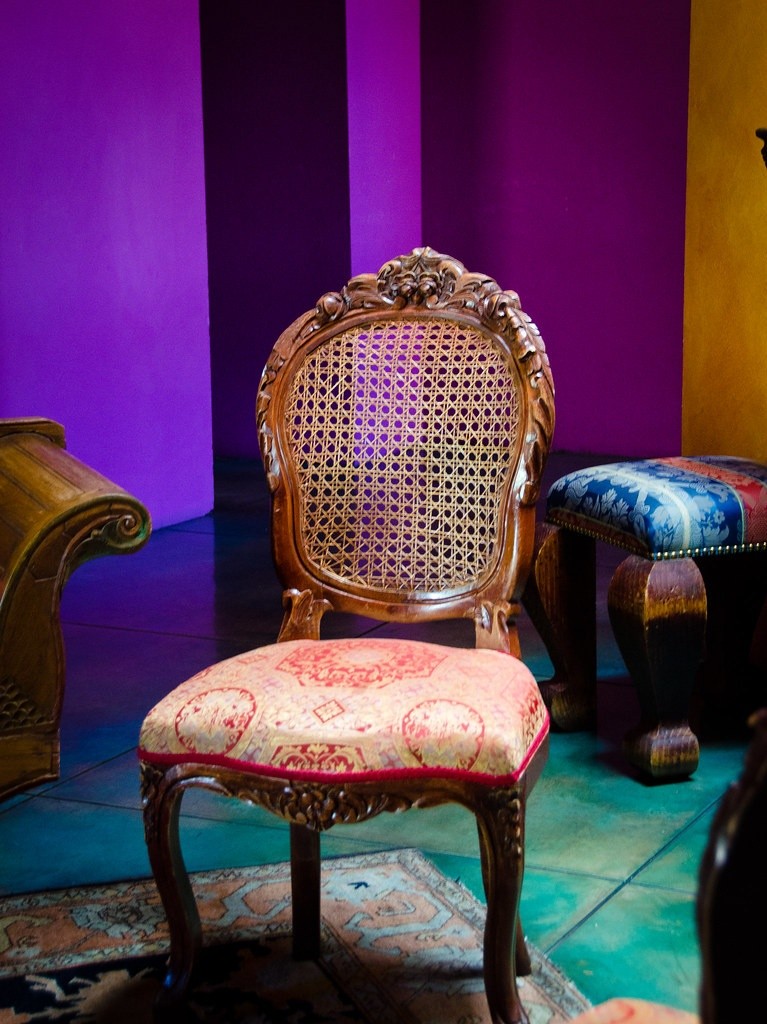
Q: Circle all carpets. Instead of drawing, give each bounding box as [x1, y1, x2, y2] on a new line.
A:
[0, 800, 597, 1024]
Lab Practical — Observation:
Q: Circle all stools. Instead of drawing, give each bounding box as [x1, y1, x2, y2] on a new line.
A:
[521, 458, 765, 783]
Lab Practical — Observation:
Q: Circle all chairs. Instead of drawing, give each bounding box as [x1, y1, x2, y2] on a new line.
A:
[1, 412, 153, 799]
[136, 244, 554, 1024]
